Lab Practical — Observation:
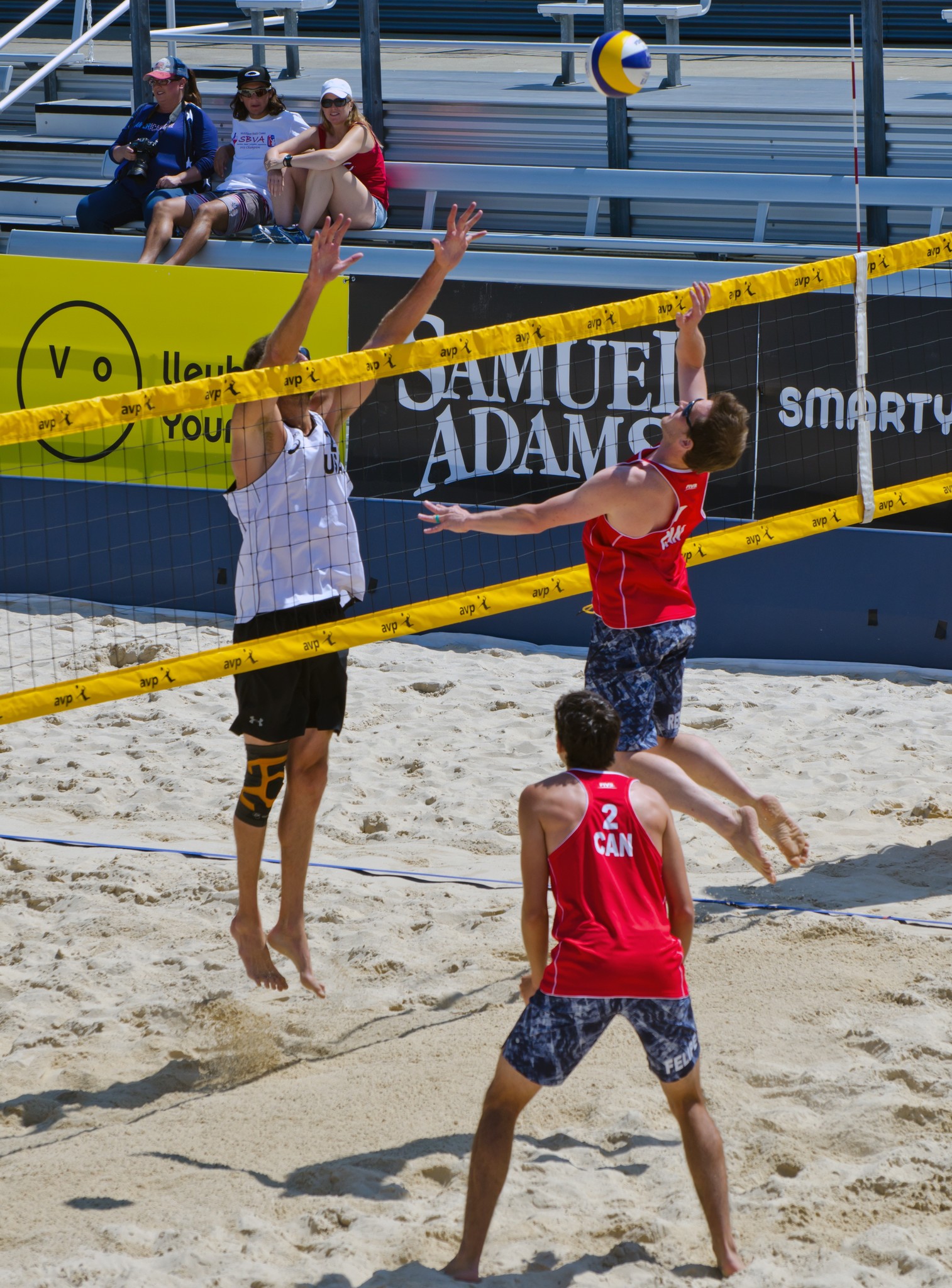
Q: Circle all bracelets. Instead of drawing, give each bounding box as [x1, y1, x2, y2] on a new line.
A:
[283, 158, 286, 167]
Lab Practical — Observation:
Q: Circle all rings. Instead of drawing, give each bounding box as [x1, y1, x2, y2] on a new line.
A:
[436, 514, 440, 524]
[266, 165, 268, 168]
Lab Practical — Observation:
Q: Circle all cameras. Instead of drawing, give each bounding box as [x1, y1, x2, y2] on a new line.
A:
[128, 138, 157, 185]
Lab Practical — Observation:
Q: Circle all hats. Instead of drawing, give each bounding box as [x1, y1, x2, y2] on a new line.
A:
[143, 55, 189, 81]
[236, 65, 272, 88]
[321, 77, 352, 101]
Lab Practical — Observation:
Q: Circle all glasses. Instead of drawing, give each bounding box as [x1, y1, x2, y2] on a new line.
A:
[321, 98, 351, 108]
[238, 86, 271, 98]
[682, 398, 703, 428]
[299, 347, 311, 360]
[148, 79, 179, 86]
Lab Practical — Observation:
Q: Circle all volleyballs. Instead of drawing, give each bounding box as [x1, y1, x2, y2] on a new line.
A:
[586, 30, 652, 99]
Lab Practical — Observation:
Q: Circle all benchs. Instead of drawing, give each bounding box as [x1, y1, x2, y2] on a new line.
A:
[536, 0, 711, 93]
[6, 160, 952, 297]
[235, 0, 337, 80]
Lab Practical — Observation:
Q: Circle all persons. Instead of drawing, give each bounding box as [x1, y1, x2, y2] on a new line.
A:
[138, 63, 312, 265]
[441, 690, 755, 1281]
[76, 56, 219, 234]
[224, 201, 488, 1001]
[264, 77, 389, 238]
[418, 280, 809, 883]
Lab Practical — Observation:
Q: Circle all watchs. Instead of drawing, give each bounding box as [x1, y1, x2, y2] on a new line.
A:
[285, 154, 293, 167]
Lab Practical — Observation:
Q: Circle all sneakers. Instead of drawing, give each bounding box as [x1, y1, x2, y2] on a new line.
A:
[252, 222, 284, 243]
[271, 223, 310, 244]
